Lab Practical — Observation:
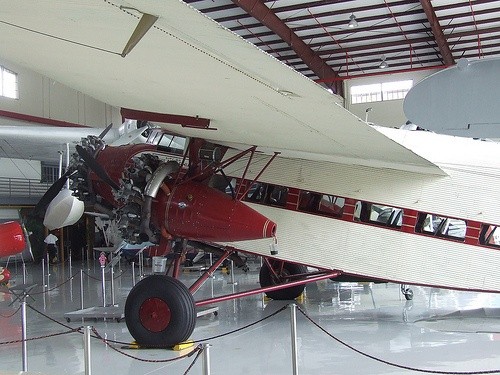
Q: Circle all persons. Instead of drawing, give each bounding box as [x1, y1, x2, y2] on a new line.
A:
[44, 233, 58, 264]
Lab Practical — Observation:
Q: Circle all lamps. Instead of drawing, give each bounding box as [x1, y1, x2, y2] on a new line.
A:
[348, 12, 358, 27]
[379, 53, 389, 69]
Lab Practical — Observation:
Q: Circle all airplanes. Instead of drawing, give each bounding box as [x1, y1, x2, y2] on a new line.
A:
[0, 0, 500, 345]
